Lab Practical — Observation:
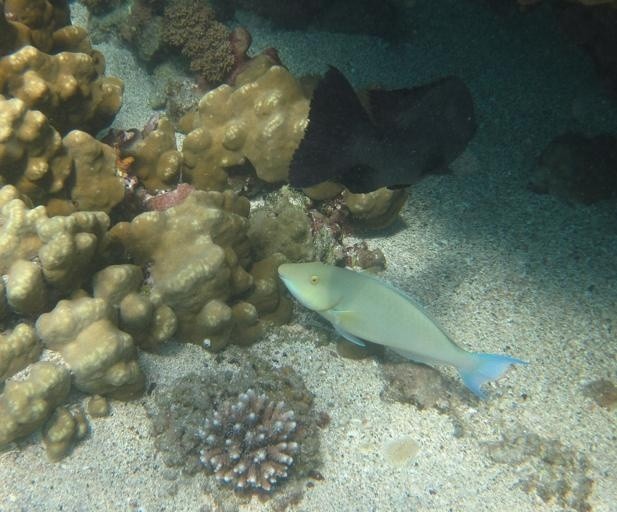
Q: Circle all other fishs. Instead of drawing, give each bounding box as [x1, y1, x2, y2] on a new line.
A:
[277, 262, 529, 400]
[288, 64, 478, 194]
